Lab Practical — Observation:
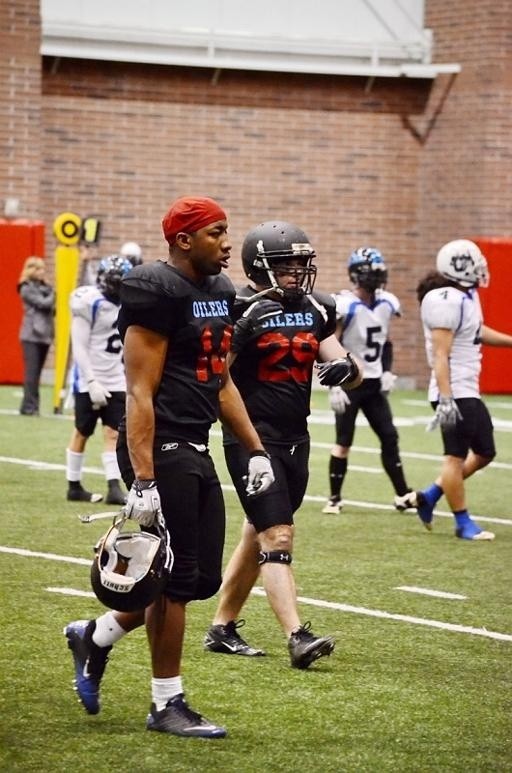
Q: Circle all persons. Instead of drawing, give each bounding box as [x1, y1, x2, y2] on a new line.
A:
[63, 196, 274, 736]
[321, 247, 420, 514]
[202, 221, 364, 670]
[415, 239, 512, 540]
[16, 238, 143, 505]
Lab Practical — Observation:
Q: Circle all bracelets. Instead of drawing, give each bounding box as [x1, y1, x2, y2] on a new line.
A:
[248, 449, 272, 460]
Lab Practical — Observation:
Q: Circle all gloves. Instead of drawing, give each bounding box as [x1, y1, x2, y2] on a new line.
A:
[431, 392, 464, 432]
[315, 352, 358, 414]
[241, 450, 276, 497]
[234, 299, 284, 332]
[379, 371, 398, 391]
[322, 500, 343, 513]
[124, 480, 166, 527]
[86, 381, 112, 407]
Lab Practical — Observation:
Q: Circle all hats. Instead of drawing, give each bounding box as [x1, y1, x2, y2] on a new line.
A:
[162, 194, 227, 246]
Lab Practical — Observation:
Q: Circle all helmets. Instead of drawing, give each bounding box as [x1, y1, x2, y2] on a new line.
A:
[436, 239, 490, 288]
[91, 516, 174, 612]
[242, 220, 316, 300]
[348, 246, 387, 292]
[97, 254, 134, 305]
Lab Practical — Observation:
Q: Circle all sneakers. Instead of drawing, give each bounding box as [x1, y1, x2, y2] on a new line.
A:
[203, 619, 267, 656]
[394, 490, 495, 540]
[145, 693, 227, 737]
[68, 480, 127, 504]
[288, 621, 335, 669]
[63, 621, 112, 715]
